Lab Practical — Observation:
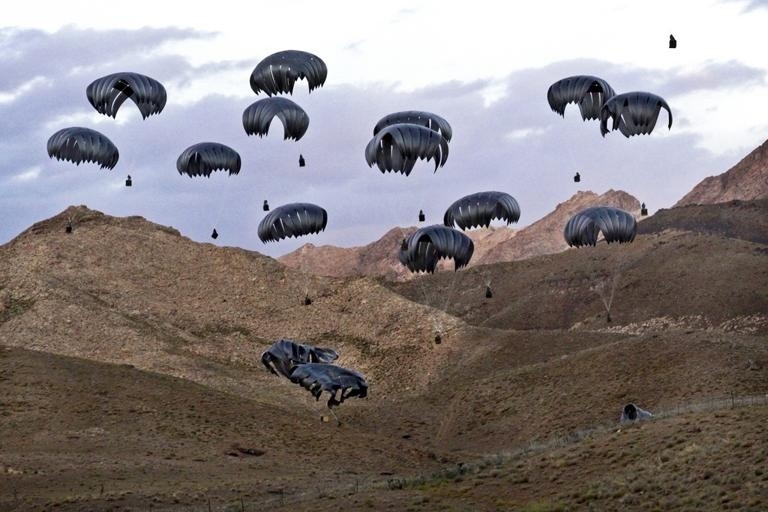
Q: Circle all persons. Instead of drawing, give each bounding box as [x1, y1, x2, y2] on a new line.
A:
[620, 402, 652, 422]
